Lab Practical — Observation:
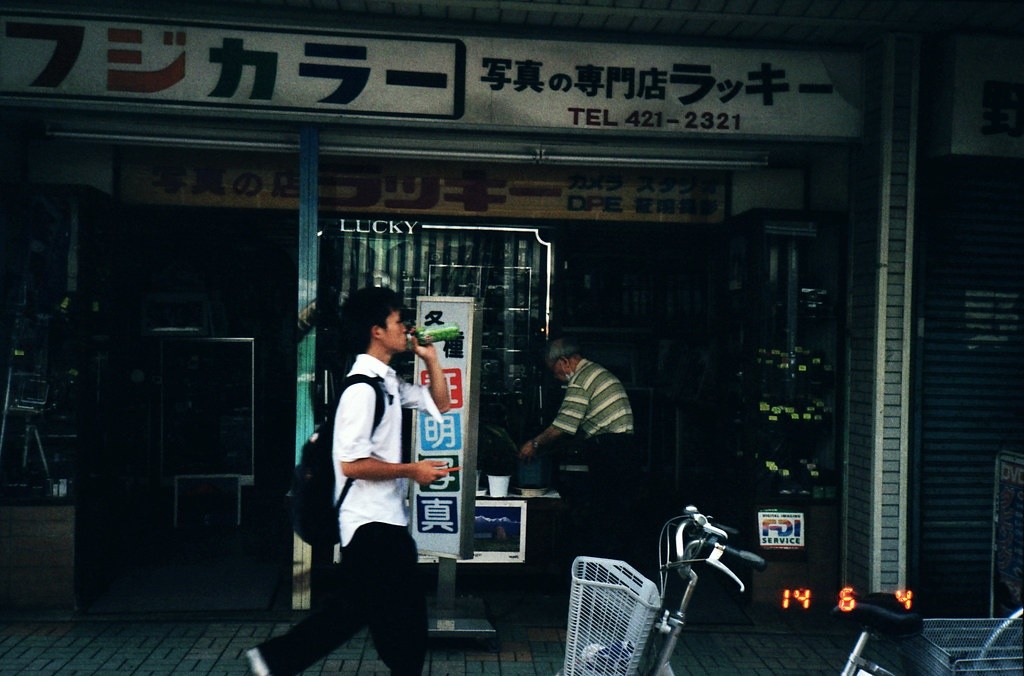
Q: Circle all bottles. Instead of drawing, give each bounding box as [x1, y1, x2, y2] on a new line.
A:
[405, 322, 462, 344]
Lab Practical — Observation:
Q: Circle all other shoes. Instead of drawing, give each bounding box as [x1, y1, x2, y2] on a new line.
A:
[246, 648, 270, 676]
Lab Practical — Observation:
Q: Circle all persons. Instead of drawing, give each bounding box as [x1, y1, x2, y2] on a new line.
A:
[518, 337, 635, 573]
[245, 287, 453, 676]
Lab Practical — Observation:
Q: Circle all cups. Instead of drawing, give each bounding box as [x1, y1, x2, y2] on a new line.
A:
[487, 475, 511, 497]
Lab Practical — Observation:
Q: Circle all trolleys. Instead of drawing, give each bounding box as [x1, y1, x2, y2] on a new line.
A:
[1, 366, 79, 506]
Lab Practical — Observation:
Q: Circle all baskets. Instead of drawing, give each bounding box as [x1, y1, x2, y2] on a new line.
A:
[563, 555, 661, 676]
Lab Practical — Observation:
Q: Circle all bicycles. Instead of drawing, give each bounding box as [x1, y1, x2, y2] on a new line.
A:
[551, 504, 1024, 676]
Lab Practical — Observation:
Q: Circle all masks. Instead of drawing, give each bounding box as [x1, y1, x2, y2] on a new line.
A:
[559, 359, 574, 381]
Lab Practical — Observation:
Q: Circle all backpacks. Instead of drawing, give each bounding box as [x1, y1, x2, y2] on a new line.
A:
[286, 374, 385, 546]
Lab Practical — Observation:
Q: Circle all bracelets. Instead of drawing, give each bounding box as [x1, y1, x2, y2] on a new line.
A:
[531, 438, 539, 452]
